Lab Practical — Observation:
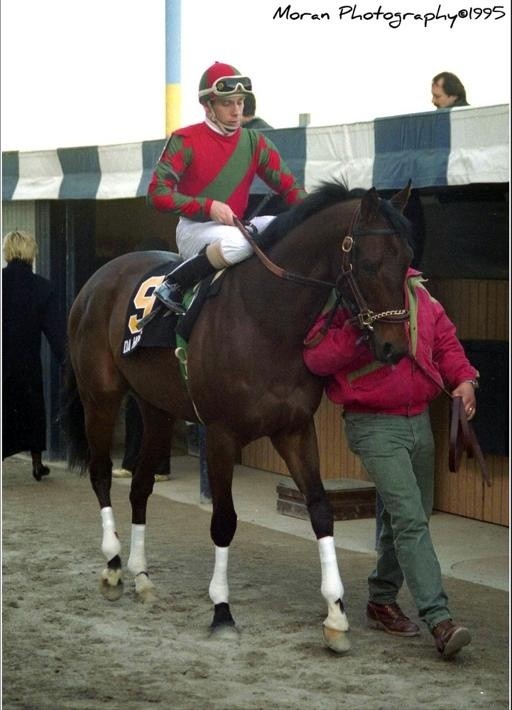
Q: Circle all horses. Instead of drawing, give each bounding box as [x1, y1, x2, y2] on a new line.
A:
[65, 173, 417, 656]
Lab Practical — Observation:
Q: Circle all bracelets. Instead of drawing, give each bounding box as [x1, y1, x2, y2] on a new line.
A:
[467, 380, 479, 389]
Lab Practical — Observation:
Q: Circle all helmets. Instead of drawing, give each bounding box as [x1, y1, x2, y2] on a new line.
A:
[196, 60, 255, 104]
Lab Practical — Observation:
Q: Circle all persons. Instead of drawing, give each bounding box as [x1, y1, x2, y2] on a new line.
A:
[145, 62, 306, 313]
[303, 266, 480, 661]
[3, 230, 70, 481]
[431, 73, 471, 110]
[240, 95, 273, 129]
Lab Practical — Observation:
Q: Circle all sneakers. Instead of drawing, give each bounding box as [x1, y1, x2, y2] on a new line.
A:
[154, 473, 172, 483]
[112, 466, 133, 479]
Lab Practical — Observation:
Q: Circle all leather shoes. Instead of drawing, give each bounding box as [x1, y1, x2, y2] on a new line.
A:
[32, 464, 51, 482]
[428, 617, 473, 661]
[365, 598, 422, 638]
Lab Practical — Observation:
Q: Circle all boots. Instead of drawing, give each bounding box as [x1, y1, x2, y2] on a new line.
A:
[152, 242, 223, 316]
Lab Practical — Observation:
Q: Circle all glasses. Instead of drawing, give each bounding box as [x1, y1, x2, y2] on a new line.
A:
[211, 76, 253, 98]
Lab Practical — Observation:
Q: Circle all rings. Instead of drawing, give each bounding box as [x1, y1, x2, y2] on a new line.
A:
[470, 408, 473, 412]
